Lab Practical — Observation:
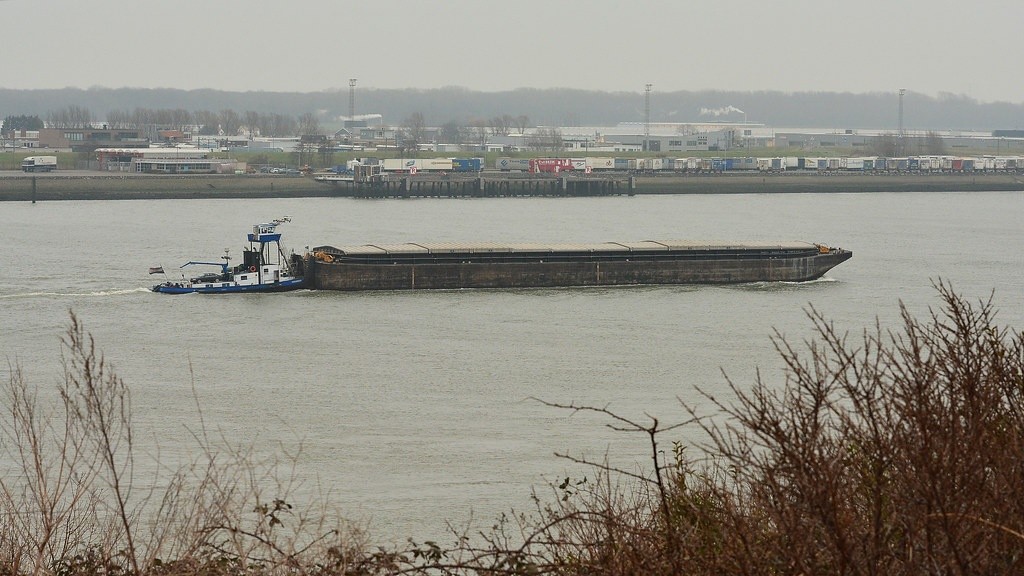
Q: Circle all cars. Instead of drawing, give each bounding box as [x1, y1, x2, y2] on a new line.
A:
[247, 165, 301, 175]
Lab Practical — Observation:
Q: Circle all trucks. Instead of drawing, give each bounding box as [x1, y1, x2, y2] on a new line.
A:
[21, 155, 57, 173]
[528, 157, 575, 175]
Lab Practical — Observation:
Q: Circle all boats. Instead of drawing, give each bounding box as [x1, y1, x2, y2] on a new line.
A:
[153, 217, 306, 296]
[286, 241, 854, 292]
